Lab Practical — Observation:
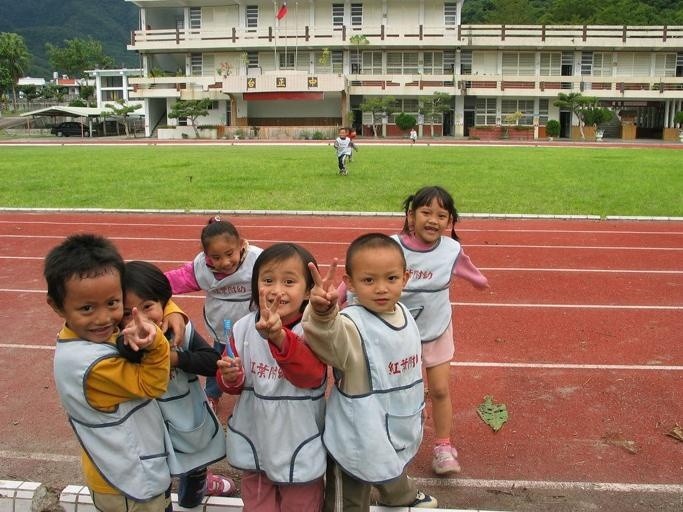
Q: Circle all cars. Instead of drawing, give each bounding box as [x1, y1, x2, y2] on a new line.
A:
[50, 121, 97, 138]
[93, 120, 125, 133]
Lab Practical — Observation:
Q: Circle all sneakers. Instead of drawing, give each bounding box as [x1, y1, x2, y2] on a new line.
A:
[379, 489, 438, 508]
[432, 446, 461, 474]
[204, 471, 236, 495]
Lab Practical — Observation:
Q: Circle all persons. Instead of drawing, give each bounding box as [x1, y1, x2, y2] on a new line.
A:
[162, 212, 266, 417]
[115, 258, 222, 509]
[333, 128, 359, 176]
[40, 231, 174, 512]
[299, 232, 440, 511]
[213, 240, 328, 511]
[336, 185, 493, 475]
[345, 128, 358, 163]
[408, 127, 418, 143]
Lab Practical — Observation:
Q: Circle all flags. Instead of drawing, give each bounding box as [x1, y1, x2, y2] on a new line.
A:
[274, 3, 290, 22]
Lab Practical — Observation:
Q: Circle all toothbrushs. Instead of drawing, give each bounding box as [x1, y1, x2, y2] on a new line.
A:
[222, 319, 235, 356]
